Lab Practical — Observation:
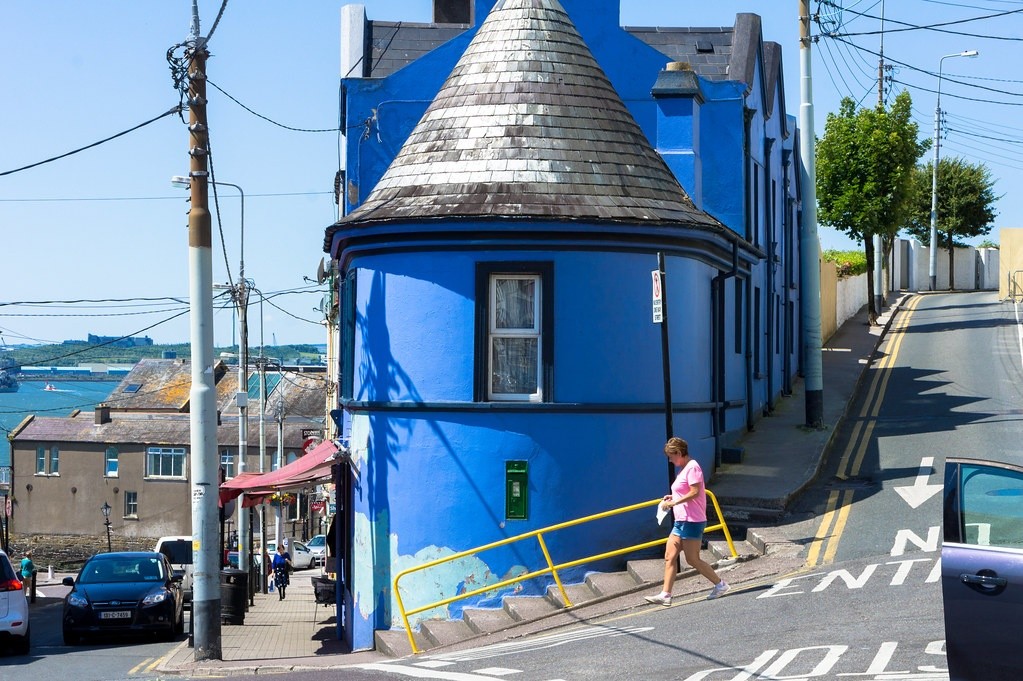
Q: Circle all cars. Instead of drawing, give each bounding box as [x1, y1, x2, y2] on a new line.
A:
[0, 548, 29, 654]
[255, 538, 315, 570]
[226, 552, 260, 596]
[60, 549, 185, 640]
[306, 535, 333, 563]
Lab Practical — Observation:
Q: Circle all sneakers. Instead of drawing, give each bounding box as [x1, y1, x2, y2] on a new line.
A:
[707, 584, 730, 600]
[645, 595, 672, 606]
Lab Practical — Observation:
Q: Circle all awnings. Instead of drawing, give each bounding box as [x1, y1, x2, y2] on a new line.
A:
[218, 438, 343, 508]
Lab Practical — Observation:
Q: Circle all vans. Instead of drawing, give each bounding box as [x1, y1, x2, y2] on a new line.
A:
[154, 536, 199, 600]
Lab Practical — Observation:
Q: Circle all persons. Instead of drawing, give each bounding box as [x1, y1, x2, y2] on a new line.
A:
[642, 435, 733, 606]
[19, 550, 34, 597]
[271, 544, 293, 601]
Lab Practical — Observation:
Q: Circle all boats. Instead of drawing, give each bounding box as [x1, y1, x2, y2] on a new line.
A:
[0, 370, 22, 393]
[44, 383, 55, 392]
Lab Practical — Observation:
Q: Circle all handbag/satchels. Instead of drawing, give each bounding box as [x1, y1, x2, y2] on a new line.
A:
[270, 578, 275, 592]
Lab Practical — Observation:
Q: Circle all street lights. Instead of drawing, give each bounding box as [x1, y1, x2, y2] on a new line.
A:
[213, 280, 270, 594]
[170, 174, 249, 574]
[927, 50, 978, 291]
[216, 349, 285, 555]
[100, 500, 114, 552]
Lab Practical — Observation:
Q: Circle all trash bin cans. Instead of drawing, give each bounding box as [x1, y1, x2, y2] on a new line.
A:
[218, 569, 249, 626]
